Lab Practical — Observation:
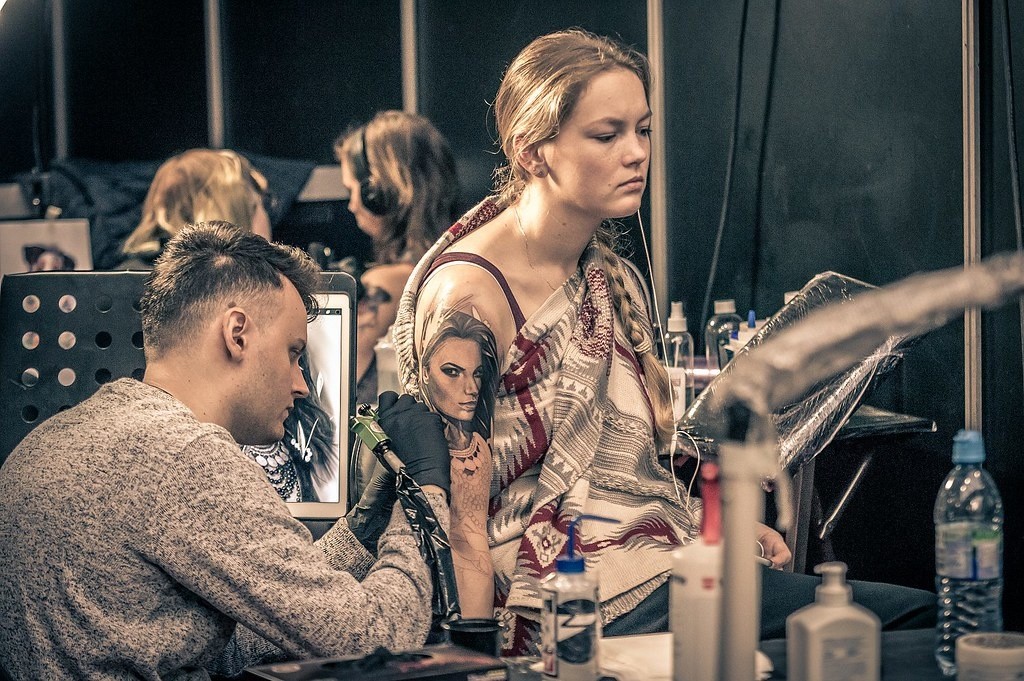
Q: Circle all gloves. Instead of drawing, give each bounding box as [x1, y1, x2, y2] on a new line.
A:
[375, 390, 453, 508]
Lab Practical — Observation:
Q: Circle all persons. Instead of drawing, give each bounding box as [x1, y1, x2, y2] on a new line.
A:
[335, 111, 459, 407]
[394, 29, 938, 643]
[95, 150, 272, 386]
[0, 221, 448, 681]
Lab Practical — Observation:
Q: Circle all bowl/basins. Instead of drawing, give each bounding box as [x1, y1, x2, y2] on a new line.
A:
[440, 617, 500, 654]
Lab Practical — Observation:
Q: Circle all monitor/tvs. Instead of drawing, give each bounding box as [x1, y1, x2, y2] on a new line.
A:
[231, 271, 358, 526]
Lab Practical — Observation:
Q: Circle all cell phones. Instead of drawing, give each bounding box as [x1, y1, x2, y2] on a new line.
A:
[755, 553, 773, 568]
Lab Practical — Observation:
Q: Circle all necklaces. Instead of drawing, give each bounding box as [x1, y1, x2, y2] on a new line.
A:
[514, 204, 557, 293]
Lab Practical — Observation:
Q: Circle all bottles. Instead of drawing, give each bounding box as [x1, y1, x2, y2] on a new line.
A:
[670, 459, 725, 681]
[955, 632, 1023, 681]
[731, 290, 800, 356]
[786, 561, 881, 681]
[539, 514, 623, 681]
[933, 427, 1004, 676]
[705, 298, 743, 372]
[663, 300, 696, 422]
